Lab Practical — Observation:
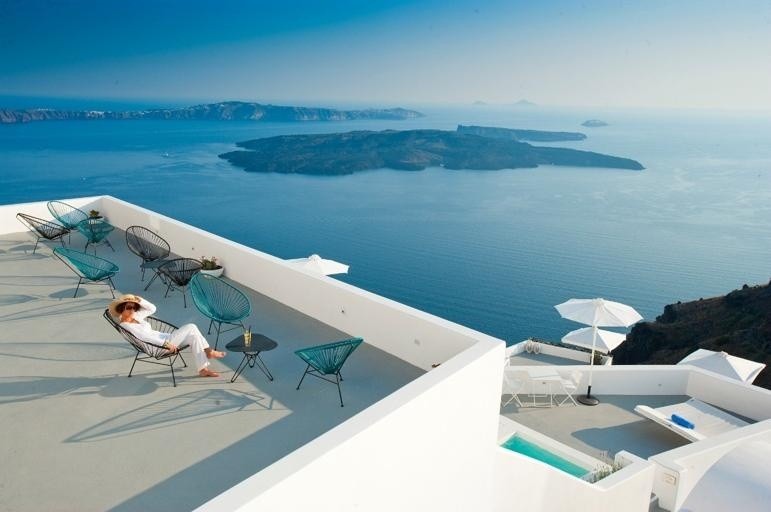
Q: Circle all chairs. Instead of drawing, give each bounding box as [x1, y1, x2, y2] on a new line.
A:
[191, 272, 252, 349]
[294, 336, 363, 407]
[15, 213, 68, 254]
[126, 223, 173, 284]
[46, 197, 87, 243]
[79, 215, 115, 257]
[53, 246, 120, 299]
[148, 256, 203, 308]
[102, 307, 191, 389]
[504, 371, 586, 410]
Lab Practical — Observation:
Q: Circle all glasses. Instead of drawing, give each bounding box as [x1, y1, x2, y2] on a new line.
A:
[126, 304, 139, 310]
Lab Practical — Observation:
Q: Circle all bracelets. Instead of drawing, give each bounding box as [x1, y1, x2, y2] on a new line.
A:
[164, 341, 170, 347]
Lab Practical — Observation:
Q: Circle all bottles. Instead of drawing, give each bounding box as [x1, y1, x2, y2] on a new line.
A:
[671, 414, 695, 430]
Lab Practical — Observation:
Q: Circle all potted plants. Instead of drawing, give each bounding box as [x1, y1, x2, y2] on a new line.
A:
[87, 209, 103, 224]
[199, 256, 224, 279]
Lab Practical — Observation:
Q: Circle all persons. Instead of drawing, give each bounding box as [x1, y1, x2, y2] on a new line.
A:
[108, 293, 228, 378]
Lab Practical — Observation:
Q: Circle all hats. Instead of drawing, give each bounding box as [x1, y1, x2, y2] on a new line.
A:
[110, 294, 141, 316]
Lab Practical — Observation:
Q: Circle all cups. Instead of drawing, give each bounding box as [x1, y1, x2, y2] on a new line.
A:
[244, 329, 252, 347]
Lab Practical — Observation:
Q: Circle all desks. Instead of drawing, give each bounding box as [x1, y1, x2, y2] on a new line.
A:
[528, 368, 561, 408]
[139, 259, 175, 292]
[224, 333, 277, 382]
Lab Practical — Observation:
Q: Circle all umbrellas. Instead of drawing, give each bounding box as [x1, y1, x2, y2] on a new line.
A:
[284, 252, 350, 277]
[553, 298, 646, 405]
[676, 347, 768, 386]
[560, 327, 628, 355]
